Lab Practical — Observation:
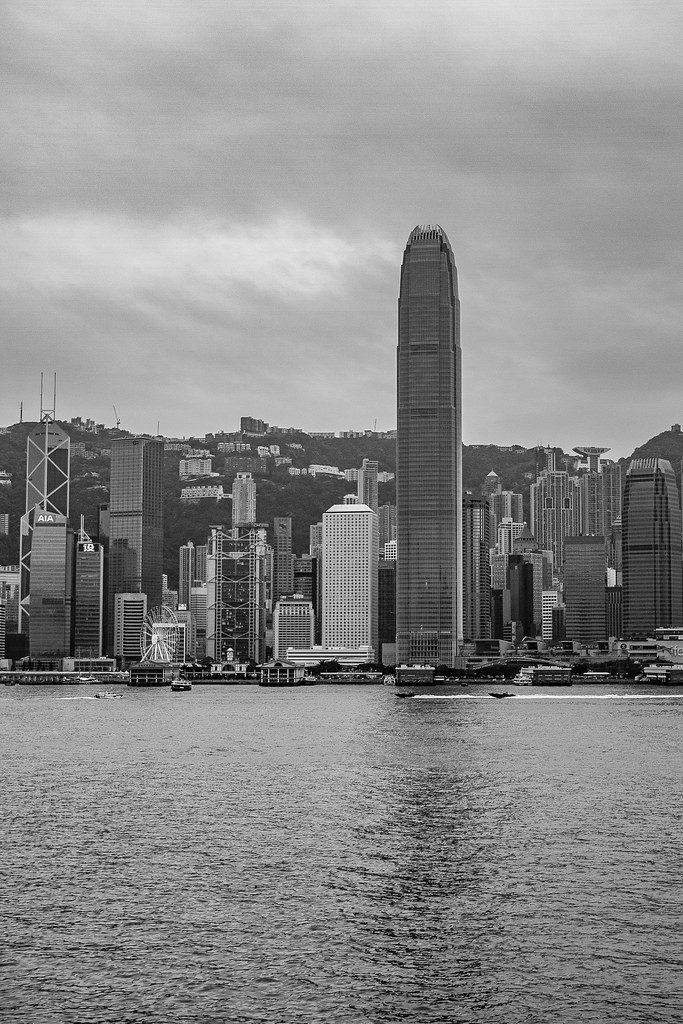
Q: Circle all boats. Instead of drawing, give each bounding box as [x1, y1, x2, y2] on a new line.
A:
[94, 691, 123, 699]
[512, 664, 571, 686]
[634, 663, 683, 682]
[171, 677, 191, 691]
[489, 692, 516, 699]
[395, 693, 421, 699]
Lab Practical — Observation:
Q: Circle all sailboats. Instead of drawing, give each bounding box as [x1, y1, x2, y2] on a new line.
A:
[61, 646, 102, 684]
